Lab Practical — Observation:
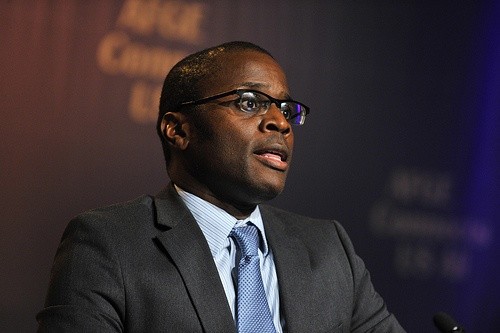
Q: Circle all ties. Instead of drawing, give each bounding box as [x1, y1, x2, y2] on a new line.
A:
[230, 226, 277, 333]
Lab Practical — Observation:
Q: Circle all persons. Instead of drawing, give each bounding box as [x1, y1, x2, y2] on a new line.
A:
[36, 41, 415, 333]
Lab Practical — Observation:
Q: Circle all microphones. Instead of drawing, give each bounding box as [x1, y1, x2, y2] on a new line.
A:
[432, 312, 467, 333]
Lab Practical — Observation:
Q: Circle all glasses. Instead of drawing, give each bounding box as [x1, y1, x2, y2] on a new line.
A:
[170, 89, 310, 126]
